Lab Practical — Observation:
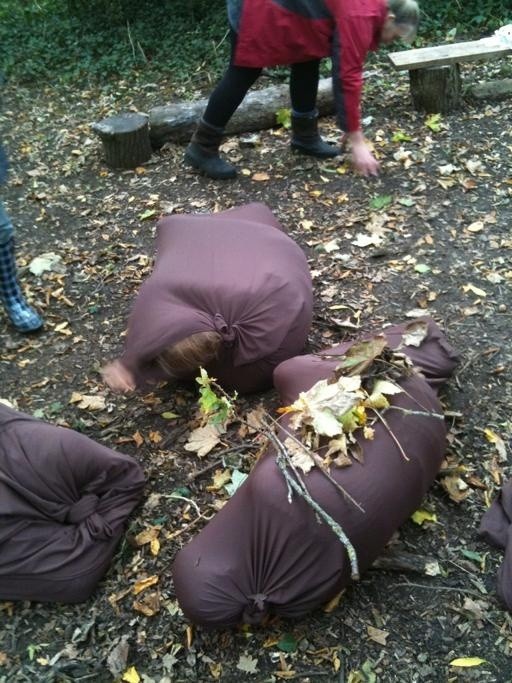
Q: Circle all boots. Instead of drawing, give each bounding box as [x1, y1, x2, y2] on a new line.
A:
[290, 106, 339, 158]
[1, 236, 44, 334]
[183, 115, 236, 178]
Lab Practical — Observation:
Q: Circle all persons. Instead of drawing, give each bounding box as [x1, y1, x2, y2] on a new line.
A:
[184, 0, 420, 180]
[0, 140, 43, 332]
